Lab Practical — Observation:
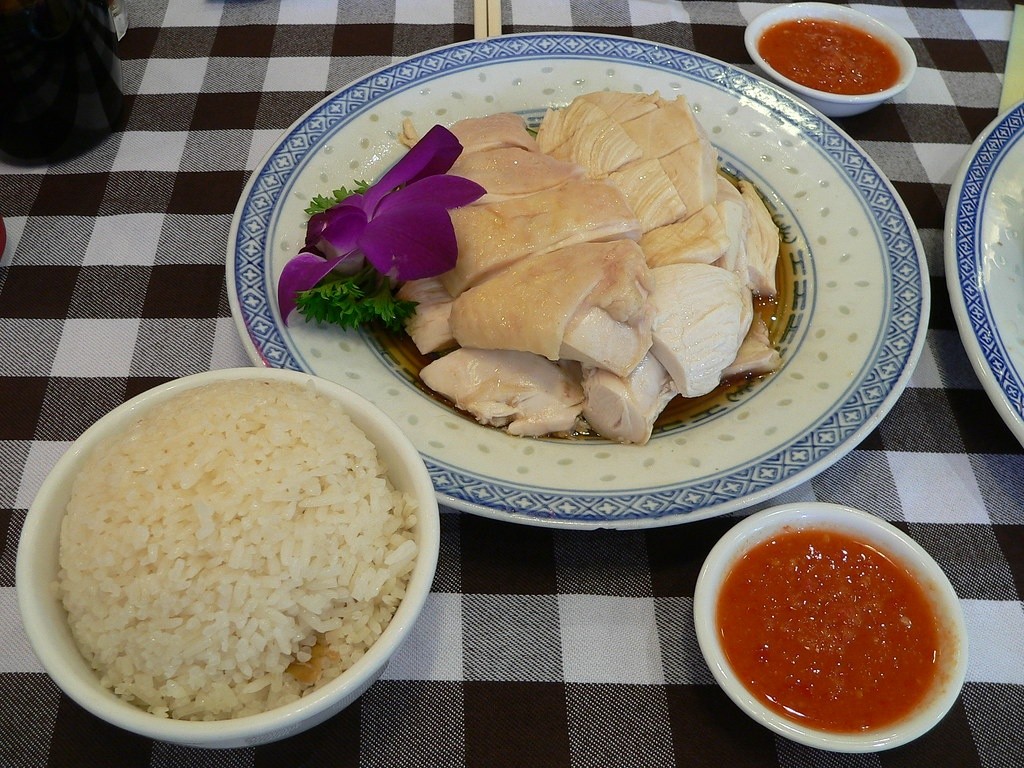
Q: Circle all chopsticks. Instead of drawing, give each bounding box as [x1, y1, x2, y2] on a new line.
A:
[473, 0, 501, 39]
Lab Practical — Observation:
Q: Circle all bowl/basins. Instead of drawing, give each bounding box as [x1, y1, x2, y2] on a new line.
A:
[693, 502, 969, 753]
[747, 2, 916, 113]
[15, 367, 440, 752]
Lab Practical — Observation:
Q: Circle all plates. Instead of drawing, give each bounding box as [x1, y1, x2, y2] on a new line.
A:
[942, 101, 1024, 455]
[224, 32, 931, 534]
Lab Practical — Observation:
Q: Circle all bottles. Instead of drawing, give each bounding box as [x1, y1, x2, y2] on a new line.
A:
[0, 1, 125, 165]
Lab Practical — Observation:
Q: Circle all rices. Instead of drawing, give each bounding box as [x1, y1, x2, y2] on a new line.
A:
[50, 380, 419, 721]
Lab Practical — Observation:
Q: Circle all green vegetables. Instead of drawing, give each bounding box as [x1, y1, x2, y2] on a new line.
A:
[297, 180, 420, 329]
